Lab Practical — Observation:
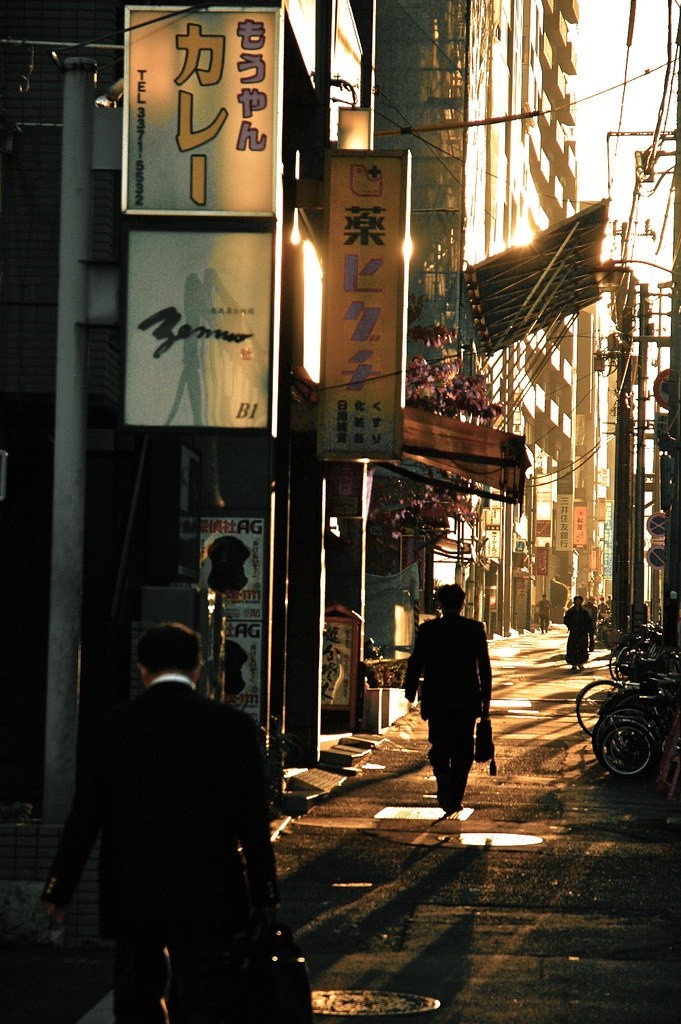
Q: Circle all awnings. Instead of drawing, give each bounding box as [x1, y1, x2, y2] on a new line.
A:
[466, 195, 612, 431]
[289, 370, 532, 517]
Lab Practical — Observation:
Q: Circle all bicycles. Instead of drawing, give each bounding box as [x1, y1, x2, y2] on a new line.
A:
[570, 620, 680, 780]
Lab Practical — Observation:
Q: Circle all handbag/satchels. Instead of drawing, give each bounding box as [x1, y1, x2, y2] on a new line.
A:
[232, 909, 314, 1024]
[475, 711, 494, 763]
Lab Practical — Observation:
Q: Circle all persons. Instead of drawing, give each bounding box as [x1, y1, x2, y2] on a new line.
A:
[563, 595, 612, 670]
[537, 594, 549, 634]
[404, 583, 491, 814]
[39, 622, 280, 1023]
